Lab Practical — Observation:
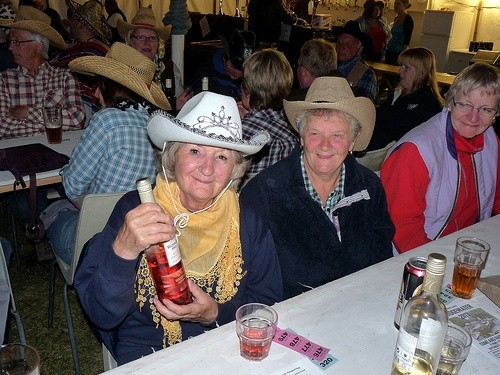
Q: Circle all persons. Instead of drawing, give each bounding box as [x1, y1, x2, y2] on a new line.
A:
[202, 28, 255, 100]
[36, 40, 173, 268]
[236, 49, 298, 195]
[73, 91, 283, 366]
[47, 0, 112, 96]
[369, 49, 448, 151]
[118, 7, 195, 120]
[0, 237, 14, 345]
[380, 62, 500, 261]
[290, 38, 340, 100]
[332, 18, 378, 103]
[384, 0, 416, 66]
[247, 0, 298, 49]
[0, 5, 85, 233]
[359, 0, 391, 61]
[240, 75, 397, 298]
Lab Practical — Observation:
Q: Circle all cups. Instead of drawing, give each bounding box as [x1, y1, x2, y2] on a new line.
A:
[451, 236, 491, 300]
[42, 105, 63, 144]
[436, 322, 473, 375]
[469, 41, 493, 52]
[235, 302, 278, 362]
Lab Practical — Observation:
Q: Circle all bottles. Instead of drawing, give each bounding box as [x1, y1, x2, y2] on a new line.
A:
[164, 80, 176, 119]
[202, 76, 208, 91]
[390, 252, 449, 375]
[136, 177, 191, 307]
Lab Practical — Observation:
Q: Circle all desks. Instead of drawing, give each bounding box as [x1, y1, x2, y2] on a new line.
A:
[97, 214, 500, 375]
[0, 129, 86, 192]
[366, 60, 457, 85]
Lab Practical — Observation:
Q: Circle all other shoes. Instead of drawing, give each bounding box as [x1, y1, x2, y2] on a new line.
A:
[23, 219, 45, 243]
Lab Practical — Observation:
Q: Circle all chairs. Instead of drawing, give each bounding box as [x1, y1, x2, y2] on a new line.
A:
[48, 192, 129, 375]
[355, 140, 396, 171]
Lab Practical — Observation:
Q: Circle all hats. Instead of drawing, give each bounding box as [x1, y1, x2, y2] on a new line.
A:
[0, 5, 67, 51]
[228, 30, 262, 70]
[117, 7, 172, 42]
[363, 0, 384, 19]
[146, 91, 270, 156]
[332, 21, 372, 54]
[68, 42, 172, 111]
[283, 77, 376, 151]
[43, 8, 68, 35]
[0, 1, 16, 34]
[66, 0, 113, 40]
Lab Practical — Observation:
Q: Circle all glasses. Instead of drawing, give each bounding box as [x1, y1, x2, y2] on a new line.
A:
[5, 38, 44, 46]
[294, 64, 310, 74]
[130, 34, 157, 41]
[453, 96, 497, 117]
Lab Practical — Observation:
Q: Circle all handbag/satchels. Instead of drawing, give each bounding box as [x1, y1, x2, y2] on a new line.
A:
[0, 143, 70, 180]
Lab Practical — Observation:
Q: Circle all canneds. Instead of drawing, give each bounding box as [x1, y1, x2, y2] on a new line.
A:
[394, 257, 428, 330]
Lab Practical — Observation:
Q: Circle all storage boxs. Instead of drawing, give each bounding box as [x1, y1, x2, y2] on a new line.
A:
[312, 14, 332, 28]
